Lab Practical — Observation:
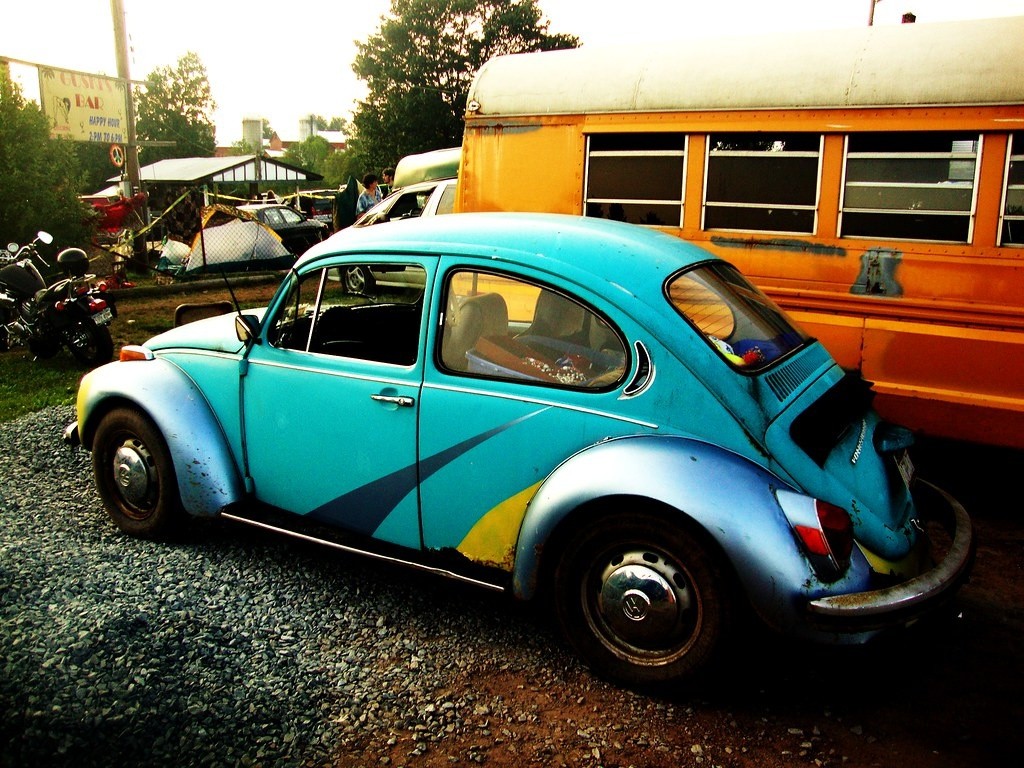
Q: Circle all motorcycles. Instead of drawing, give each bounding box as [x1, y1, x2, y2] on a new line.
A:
[0, 230, 118, 367]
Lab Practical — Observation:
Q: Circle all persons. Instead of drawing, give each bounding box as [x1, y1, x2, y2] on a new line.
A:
[382, 168, 395, 198]
[355, 174, 382, 219]
[162, 184, 278, 244]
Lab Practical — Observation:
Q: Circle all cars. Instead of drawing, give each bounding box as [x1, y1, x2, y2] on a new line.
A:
[332, 148, 464, 296]
[239, 204, 330, 250]
[66, 212, 974, 694]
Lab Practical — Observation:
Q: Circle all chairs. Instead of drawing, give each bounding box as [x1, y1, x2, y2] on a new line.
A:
[442, 292, 509, 371]
[513, 288, 585, 343]
